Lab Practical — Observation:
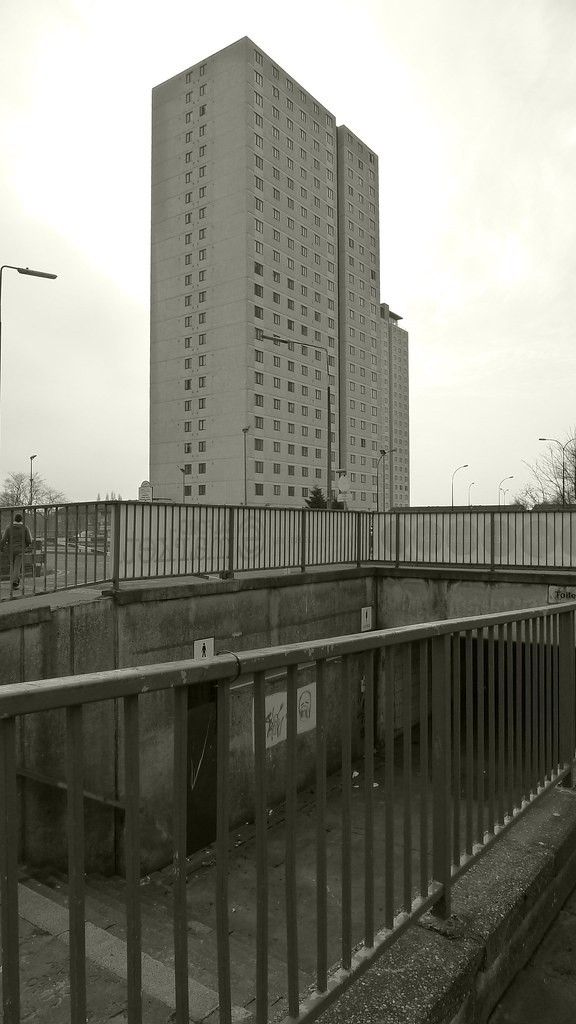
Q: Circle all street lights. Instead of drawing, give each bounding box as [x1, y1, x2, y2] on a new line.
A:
[241, 424, 251, 504]
[468, 482, 474, 506]
[499, 487, 509, 506]
[498, 475, 514, 506]
[0, 265, 58, 420]
[29, 455, 38, 509]
[451, 464, 470, 510]
[259, 333, 333, 509]
[376, 447, 398, 512]
[538, 437, 576, 508]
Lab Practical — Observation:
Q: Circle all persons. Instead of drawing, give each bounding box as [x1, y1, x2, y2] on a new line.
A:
[0, 514, 33, 590]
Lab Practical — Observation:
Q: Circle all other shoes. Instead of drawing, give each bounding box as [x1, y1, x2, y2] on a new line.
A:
[13, 579, 20, 589]
